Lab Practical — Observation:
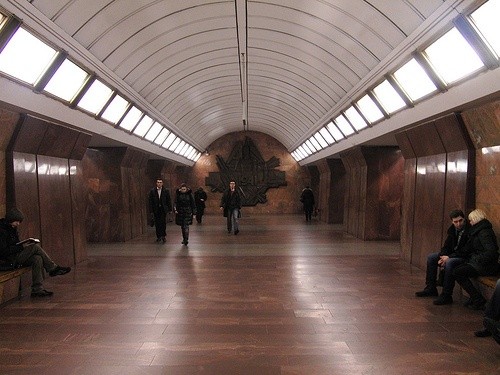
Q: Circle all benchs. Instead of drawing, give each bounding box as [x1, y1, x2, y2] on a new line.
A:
[0, 266, 32, 304]
[461, 276, 500, 301]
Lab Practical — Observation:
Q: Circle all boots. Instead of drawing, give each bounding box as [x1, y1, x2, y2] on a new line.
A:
[182, 227, 189, 246]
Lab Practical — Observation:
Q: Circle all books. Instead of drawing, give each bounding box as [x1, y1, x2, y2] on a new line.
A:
[16, 238, 40, 248]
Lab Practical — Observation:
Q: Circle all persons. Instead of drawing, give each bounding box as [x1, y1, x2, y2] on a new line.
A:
[300, 185, 315, 224]
[416, 210, 471, 297]
[220, 179, 242, 234]
[433, 208, 500, 304]
[475, 279, 500, 338]
[173, 183, 197, 246]
[148, 178, 172, 244]
[194, 186, 207, 224]
[0, 207, 71, 297]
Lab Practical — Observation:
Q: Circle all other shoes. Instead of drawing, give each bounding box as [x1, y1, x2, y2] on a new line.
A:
[416, 287, 438, 297]
[156, 238, 161, 242]
[474, 327, 491, 337]
[463, 297, 487, 309]
[30, 289, 54, 297]
[433, 297, 453, 305]
[49, 265, 71, 277]
[234, 230, 239, 235]
[483, 317, 500, 345]
[163, 236, 167, 242]
